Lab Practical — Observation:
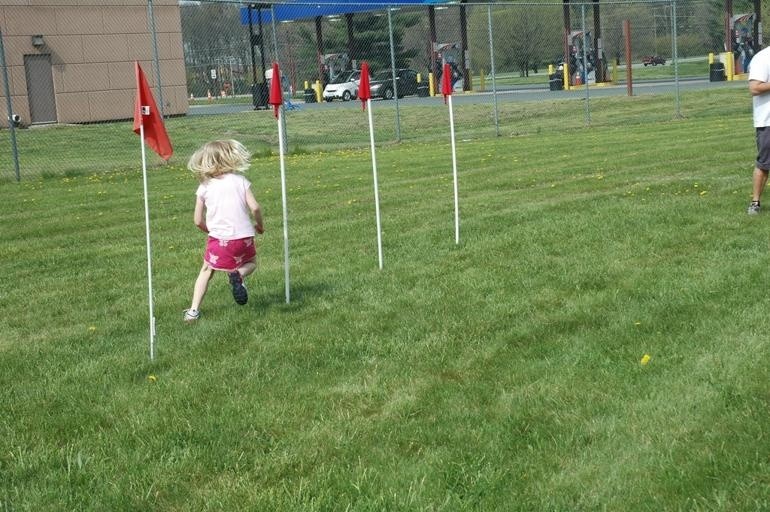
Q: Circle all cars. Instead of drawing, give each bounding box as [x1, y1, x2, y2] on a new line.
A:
[322, 70, 429, 102]
[644, 56, 666, 65]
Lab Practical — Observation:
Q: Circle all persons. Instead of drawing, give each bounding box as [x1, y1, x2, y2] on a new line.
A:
[747, 46, 770, 215]
[184, 139, 265, 321]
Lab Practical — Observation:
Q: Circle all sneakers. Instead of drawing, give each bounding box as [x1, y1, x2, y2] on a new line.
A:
[229, 270, 248, 305]
[183, 309, 200, 321]
[749, 201, 760, 215]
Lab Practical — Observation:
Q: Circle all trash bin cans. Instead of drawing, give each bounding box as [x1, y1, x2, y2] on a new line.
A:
[710, 63, 724, 82]
[417, 81, 429, 97]
[304, 89, 316, 103]
[549, 74, 562, 90]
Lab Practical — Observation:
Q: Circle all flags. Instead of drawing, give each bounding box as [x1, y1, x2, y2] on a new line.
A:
[358, 61, 370, 110]
[442, 64, 452, 104]
[132, 60, 172, 160]
[269, 63, 282, 119]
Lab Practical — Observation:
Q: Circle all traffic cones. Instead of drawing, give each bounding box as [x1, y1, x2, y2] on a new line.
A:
[575, 71, 582, 87]
